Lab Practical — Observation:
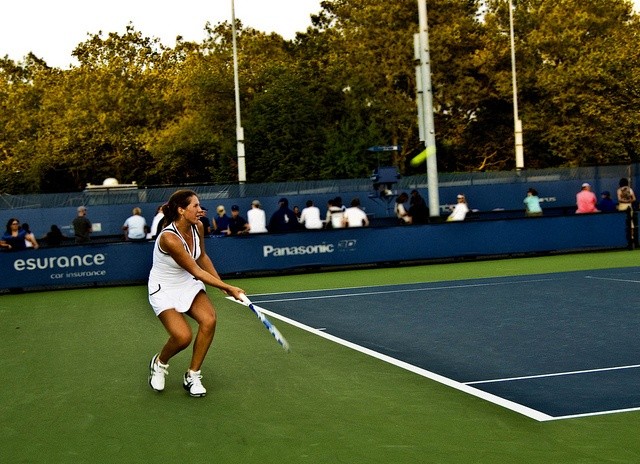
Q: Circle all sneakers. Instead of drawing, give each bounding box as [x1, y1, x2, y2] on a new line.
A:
[183, 369, 208, 398]
[147, 353, 170, 392]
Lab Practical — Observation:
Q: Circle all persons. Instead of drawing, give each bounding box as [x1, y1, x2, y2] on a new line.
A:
[523, 188, 543, 216]
[122, 206, 147, 242]
[247, 200, 269, 234]
[598, 191, 617, 212]
[574, 183, 601, 213]
[150, 206, 166, 240]
[45, 224, 68, 246]
[616, 178, 636, 240]
[21, 222, 37, 249]
[394, 191, 412, 225]
[326, 195, 347, 229]
[146, 188, 246, 398]
[71, 205, 93, 244]
[343, 199, 369, 227]
[293, 206, 301, 218]
[228, 205, 250, 235]
[446, 192, 470, 222]
[408, 189, 430, 225]
[269, 198, 298, 233]
[1, 217, 40, 250]
[328, 199, 334, 209]
[212, 205, 231, 236]
[298, 200, 324, 230]
[198, 207, 212, 235]
[144, 226, 153, 240]
